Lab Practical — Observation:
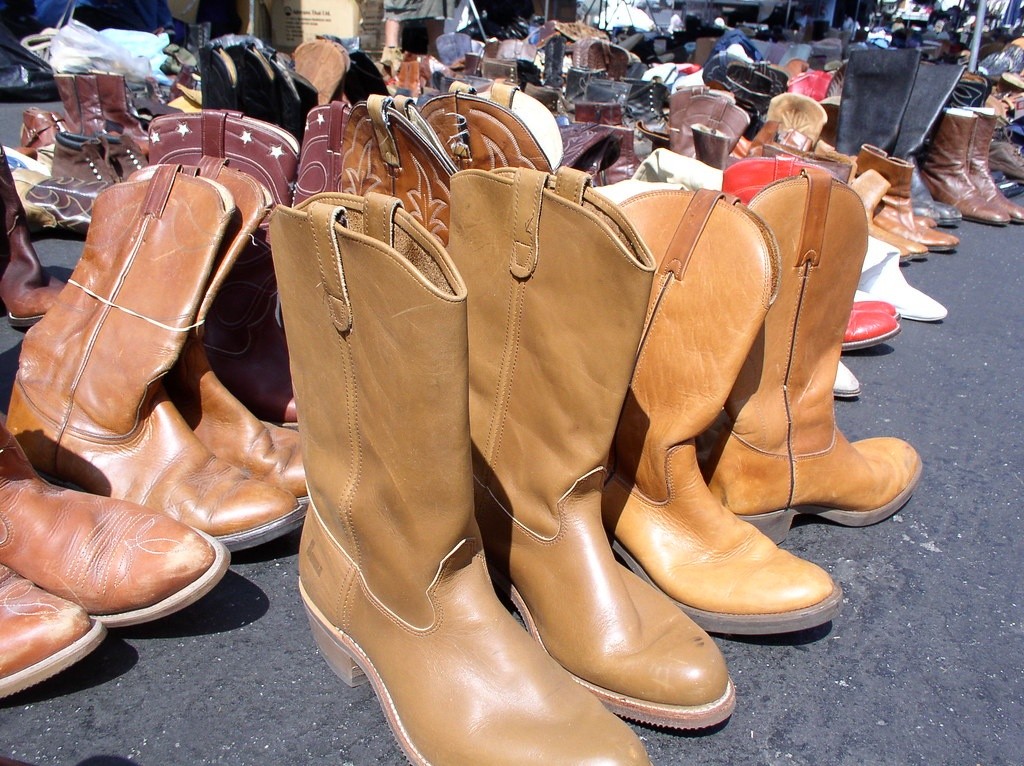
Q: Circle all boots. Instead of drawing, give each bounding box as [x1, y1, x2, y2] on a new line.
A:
[0, 36, 1024, 765]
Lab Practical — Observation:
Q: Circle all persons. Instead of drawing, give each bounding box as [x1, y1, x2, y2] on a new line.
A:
[381, 0, 543, 55]
[0, 0, 185, 47]
